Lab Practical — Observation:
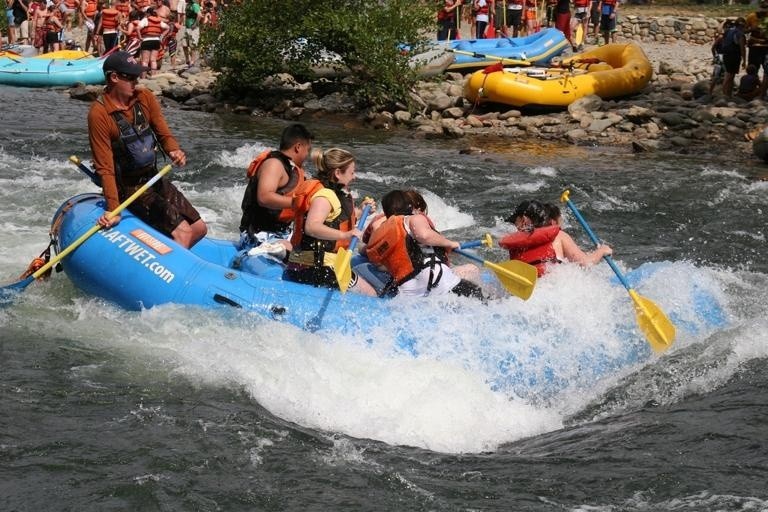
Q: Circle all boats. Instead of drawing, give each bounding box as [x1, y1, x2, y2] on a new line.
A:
[0, 49, 113, 87]
[398, 30, 575, 77]
[277, 36, 455, 90]
[54, 194, 726, 399]
[462, 42, 654, 109]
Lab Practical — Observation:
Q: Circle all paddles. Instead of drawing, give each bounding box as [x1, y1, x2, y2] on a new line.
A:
[330, 197, 377, 295]
[503, 1, 508, 37]
[559, 190, 677, 353]
[535, 0, 545, 32]
[456, 6, 461, 39]
[452, 247, 538, 301]
[0, 153, 186, 307]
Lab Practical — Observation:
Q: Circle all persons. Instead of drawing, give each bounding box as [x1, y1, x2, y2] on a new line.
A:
[90, 52, 208, 255]
[368, 189, 485, 300]
[500, 198, 613, 279]
[363, 192, 453, 269]
[434, 1, 618, 52]
[290, 148, 376, 298]
[546, 203, 561, 232]
[709, 1, 768, 99]
[242, 124, 314, 259]
[0, 0, 240, 79]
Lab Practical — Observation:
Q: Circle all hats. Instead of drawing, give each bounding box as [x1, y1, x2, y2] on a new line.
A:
[102, 51, 151, 75]
[504, 200, 545, 224]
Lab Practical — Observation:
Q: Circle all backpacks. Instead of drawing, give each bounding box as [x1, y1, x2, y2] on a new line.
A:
[717, 29, 737, 56]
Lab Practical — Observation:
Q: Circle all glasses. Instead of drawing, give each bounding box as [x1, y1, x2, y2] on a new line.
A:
[117, 71, 138, 81]
